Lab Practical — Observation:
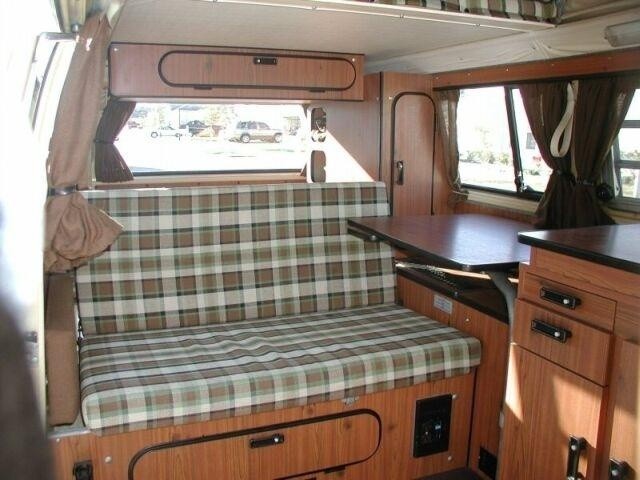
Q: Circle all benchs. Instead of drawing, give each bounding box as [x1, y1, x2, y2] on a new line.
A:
[43, 181, 482, 435]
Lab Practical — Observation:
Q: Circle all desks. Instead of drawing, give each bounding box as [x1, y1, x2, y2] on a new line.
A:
[346, 212, 542, 356]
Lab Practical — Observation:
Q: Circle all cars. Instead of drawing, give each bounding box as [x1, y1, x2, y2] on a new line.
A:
[522, 127, 544, 175]
[150, 119, 283, 143]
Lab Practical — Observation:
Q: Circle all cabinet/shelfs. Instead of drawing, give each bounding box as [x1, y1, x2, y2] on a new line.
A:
[496, 223, 639, 479]
